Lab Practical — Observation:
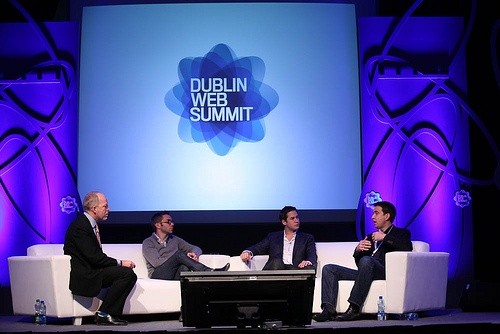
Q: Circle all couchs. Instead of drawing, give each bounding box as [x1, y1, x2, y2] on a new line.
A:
[233, 241, 450, 320]
[8, 242, 231, 325]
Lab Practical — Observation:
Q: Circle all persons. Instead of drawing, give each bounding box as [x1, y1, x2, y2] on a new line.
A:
[64, 192, 137, 325]
[312, 201, 413, 322]
[240, 206, 317, 271]
[142, 212, 230, 321]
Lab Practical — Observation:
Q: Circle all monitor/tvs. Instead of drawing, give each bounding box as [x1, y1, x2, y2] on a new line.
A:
[180, 269, 317, 329]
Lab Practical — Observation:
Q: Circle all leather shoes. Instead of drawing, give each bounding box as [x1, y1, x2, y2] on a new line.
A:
[336, 305, 362, 321]
[94, 311, 129, 326]
[311, 308, 338, 322]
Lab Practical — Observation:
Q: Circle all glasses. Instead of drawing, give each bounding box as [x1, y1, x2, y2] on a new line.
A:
[162, 220, 173, 225]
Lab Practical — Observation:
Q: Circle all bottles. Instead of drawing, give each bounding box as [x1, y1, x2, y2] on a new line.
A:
[377, 296, 386, 321]
[400, 313, 418, 321]
[35, 300, 46, 328]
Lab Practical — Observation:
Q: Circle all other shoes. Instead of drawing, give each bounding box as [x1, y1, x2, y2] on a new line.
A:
[212, 262, 231, 271]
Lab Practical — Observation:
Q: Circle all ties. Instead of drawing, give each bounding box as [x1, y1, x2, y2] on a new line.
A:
[95, 224, 102, 248]
